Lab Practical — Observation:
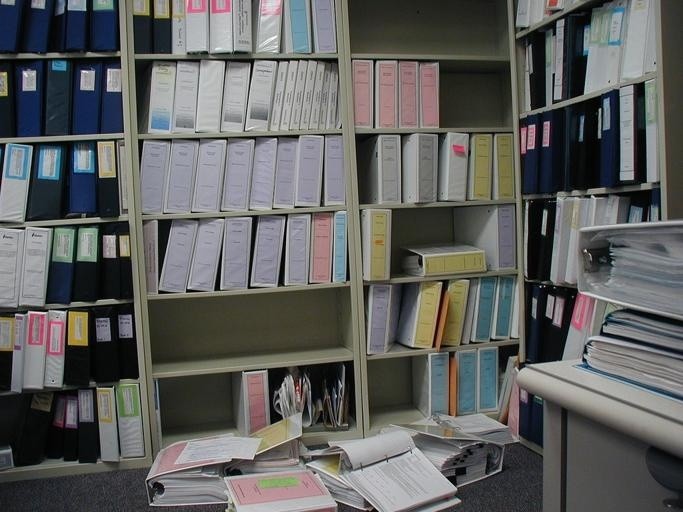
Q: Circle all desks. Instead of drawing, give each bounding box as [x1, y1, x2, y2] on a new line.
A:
[515, 359, 682, 512]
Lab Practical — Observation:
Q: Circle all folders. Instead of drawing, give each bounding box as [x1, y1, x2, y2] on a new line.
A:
[72, 224, 103, 301]
[304, 426, 461, 512]
[249, 137, 277, 210]
[547, 0, 565, 11]
[522, 115, 542, 192]
[18, 227, 53, 307]
[494, 274, 517, 338]
[512, 285, 518, 341]
[306, 457, 374, 512]
[412, 352, 451, 419]
[78, 390, 101, 463]
[138, 139, 171, 214]
[544, 29, 556, 105]
[494, 132, 514, 199]
[635, 88, 648, 183]
[331, 211, 349, 282]
[185, 218, 225, 291]
[374, 60, 398, 129]
[151, 1, 172, 54]
[351, 59, 376, 129]
[87, 0, 120, 54]
[142, 63, 176, 132]
[220, 136, 254, 210]
[390, 421, 505, 489]
[66, 0, 87, 51]
[478, 348, 500, 412]
[576, 13, 593, 96]
[402, 245, 486, 276]
[20, 311, 48, 390]
[44, 60, 72, 135]
[248, 217, 288, 289]
[516, 0, 531, 28]
[246, 58, 277, 134]
[398, 282, 443, 348]
[195, 60, 226, 132]
[472, 276, 498, 342]
[0, 0, 24, 53]
[366, 135, 403, 206]
[0, 443, 14, 469]
[102, 63, 124, 134]
[100, 224, 119, 299]
[620, 85, 634, 183]
[66, 307, 92, 384]
[172, 63, 199, 134]
[14, 389, 55, 465]
[159, 219, 196, 294]
[141, 220, 159, 295]
[581, 298, 605, 339]
[508, 372, 521, 435]
[576, 221, 683, 321]
[13, 63, 42, 137]
[232, 0, 253, 52]
[324, 132, 346, 206]
[514, 39, 525, 115]
[45, 226, 78, 305]
[522, 199, 542, 279]
[608, 0, 629, 87]
[575, 103, 591, 190]
[649, 189, 661, 221]
[447, 356, 458, 415]
[584, 194, 605, 224]
[525, 283, 544, 362]
[604, 301, 620, 319]
[49, 0, 67, 52]
[311, 1, 338, 54]
[0, 226, 24, 310]
[549, 198, 572, 284]
[564, 198, 588, 285]
[366, 284, 392, 354]
[116, 138, 127, 218]
[620, 0, 645, 80]
[411, 414, 520, 446]
[170, 0, 187, 55]
[553, 19, 563, 102]
[530, 1, 544, 27]
[1, 315, 16, 389]
[295, 135, 324, 206]
[592, 4, 612, 91]
[599, 309, 683, 355]
[282, 0, 312, 54]
[439, 133, 468, 201]
[89, 308, 115, 379]
[593, 103, 605, 187]
[96, 138, 121, 218]
[399, 58, 421, 130]
[131, 0, 153, 55]
[23, 0, 52, 55]
[518, 388, 531, 439]
[49, 396, 67, 457]
[222, 61, 251, 132]
[284, 215, 311, 285]
[547, 290, 575, 360]
[541, 290, 559, 360]
[528, 32, 544, 108]
[65, 141, 96, 213]
[442, 277, 470, 346]
[420, 61, 440, 128]
[69, 63, 102, 135]
[220, 470, 339, 512]
[253, 0, 282, 54]
[361, 210, 393, 280]
[225, 414, 312, 473]
[0, 144, 33, 220]
[273, 138, 298, 209]
[162, 139, 200, 215]
[145, 432, 229, 506]
[601, 90, 618, 187]
[452, 206, 516, 271]
[221, 216, 252, 289]
[185, 0, 210, 55]
[232, 370, 271, 436]
[436, 295, 451, 349]
[464, 278, 475, 343]
[626, 201, 645, 222]
[519, 118, 527, 172]
[114, 384, 146, 458]
[455, 350, 478, 412]
[645, 1, 656, 76]
[644, 80, 660, 183]
[64, 395, 79, 461]
[307, 212, 335, 283]
[118, 223, 132, 299]
[115, 309, 138, 377]
[10, 313, 27, 391]
[44, 309, 66, 389]
[562, 14, 580, 99]
[0, 60, 16, 137]
[583, 8, 604, 94]
[576, 336, 683, 402]
[468, 130, 492, 202]
[538, 114, 560, 194]
[209, 0, 235, 53]
[95, 387, 122, 464]
[529, 391, 543, 444]
[537, 203, 557, 279]
[401, 133, 437, 204]
[28, 140, 67, 220]
[601, 194, 628, 226]
[562, 293, 593, 361]
[561, 106, 576, 191]
[192, 140, 226, 213]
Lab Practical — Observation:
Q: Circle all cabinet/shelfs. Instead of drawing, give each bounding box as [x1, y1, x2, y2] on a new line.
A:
[341, 1, 525, 439]
[514, 1, 668, 456]
[128, 0, 364, 461]
[0, 0, 153, 483]
